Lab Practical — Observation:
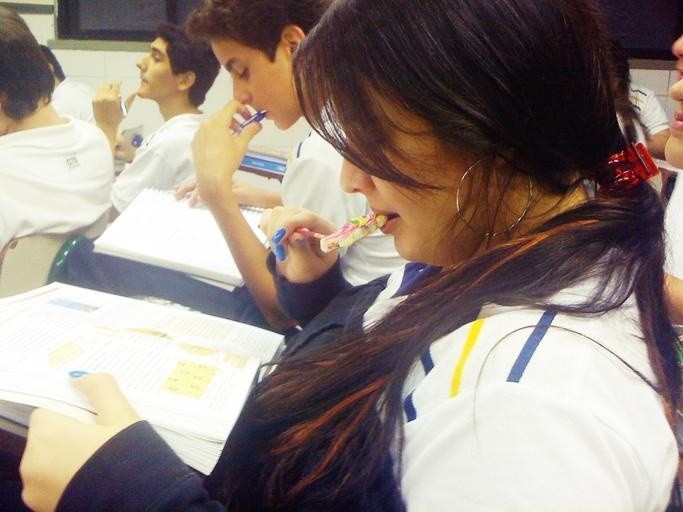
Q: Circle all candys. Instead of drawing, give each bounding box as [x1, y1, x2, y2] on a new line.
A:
[292, 213, 388, 254]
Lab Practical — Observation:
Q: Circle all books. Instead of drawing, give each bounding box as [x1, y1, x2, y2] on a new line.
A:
[89, 187, 278, 295]
[0, 279, 287, 485]
[239, 146, 292, 178]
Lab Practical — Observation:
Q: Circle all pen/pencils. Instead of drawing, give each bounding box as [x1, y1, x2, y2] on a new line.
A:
[233, 110, 267, 136]
[120, 97, 126, 119]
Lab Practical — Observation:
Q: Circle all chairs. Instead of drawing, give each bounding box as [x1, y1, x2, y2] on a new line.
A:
[0, 209, 111, 297]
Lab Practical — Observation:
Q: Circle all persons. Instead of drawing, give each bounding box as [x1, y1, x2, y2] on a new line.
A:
[178, 0, 406, 335]
[0, 4, 119, 258]
[14, 0, 681, 512]
[36, 42, 95, 130]
[658, 78, 682, 172]
[657, 30, 682, 328]
[596, 21, 671, 163]
[89, 21, 221, 224]
